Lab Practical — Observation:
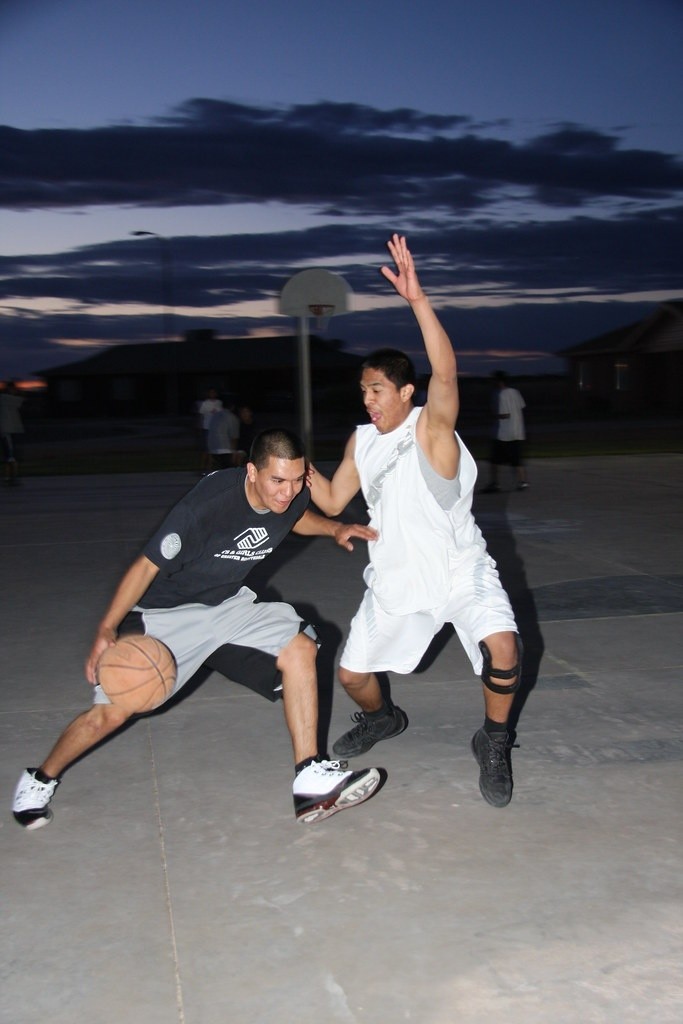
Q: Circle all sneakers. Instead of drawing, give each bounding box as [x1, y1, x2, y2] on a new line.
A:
[293, 760, 380, 824]
[13, 767, 59, 830]
[471, 728, 520, 807]
[332, 705, 408, 760]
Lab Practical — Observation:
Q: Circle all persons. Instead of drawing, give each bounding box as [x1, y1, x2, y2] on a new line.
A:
[197, 385, 259, 475]
[11, 429, 381, 832]
[479, 368, 530, 494]
[1, 382, 31, 486]
[305, 232, 526, 809]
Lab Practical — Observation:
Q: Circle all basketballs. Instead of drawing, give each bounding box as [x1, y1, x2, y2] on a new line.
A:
[98, 634, 179, 714]
